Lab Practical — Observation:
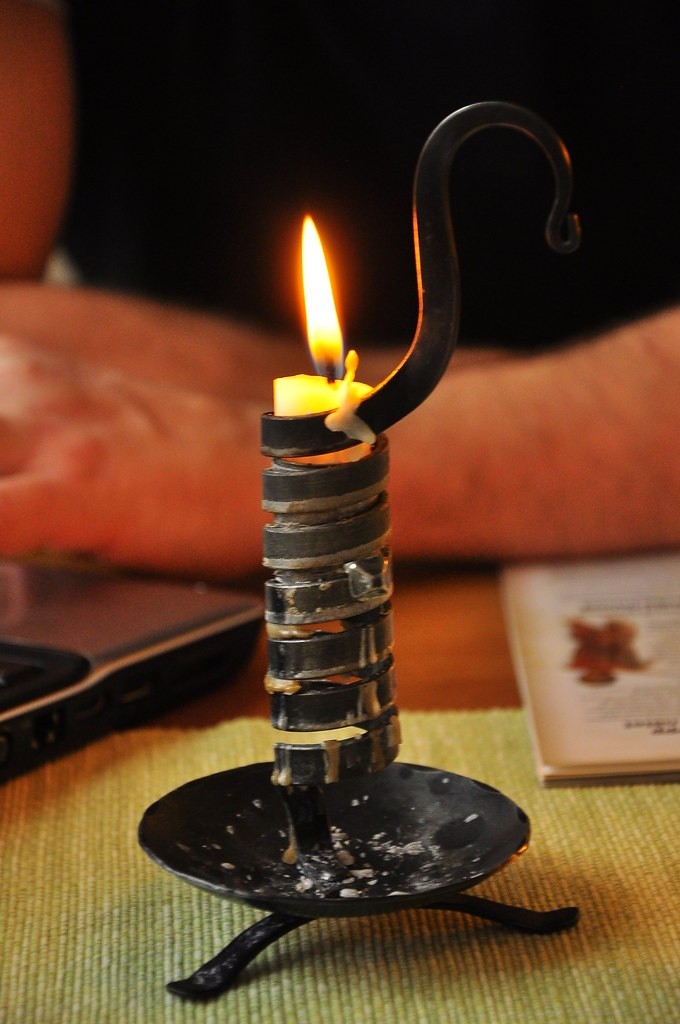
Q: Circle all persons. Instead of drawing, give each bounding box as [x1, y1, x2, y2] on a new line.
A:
[0, 0, 680, 585]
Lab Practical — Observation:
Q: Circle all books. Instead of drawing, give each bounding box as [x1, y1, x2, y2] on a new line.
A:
[495, 544, 680, 787]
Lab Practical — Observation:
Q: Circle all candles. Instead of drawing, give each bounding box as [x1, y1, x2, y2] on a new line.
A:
[272, 213, 376, 527]
